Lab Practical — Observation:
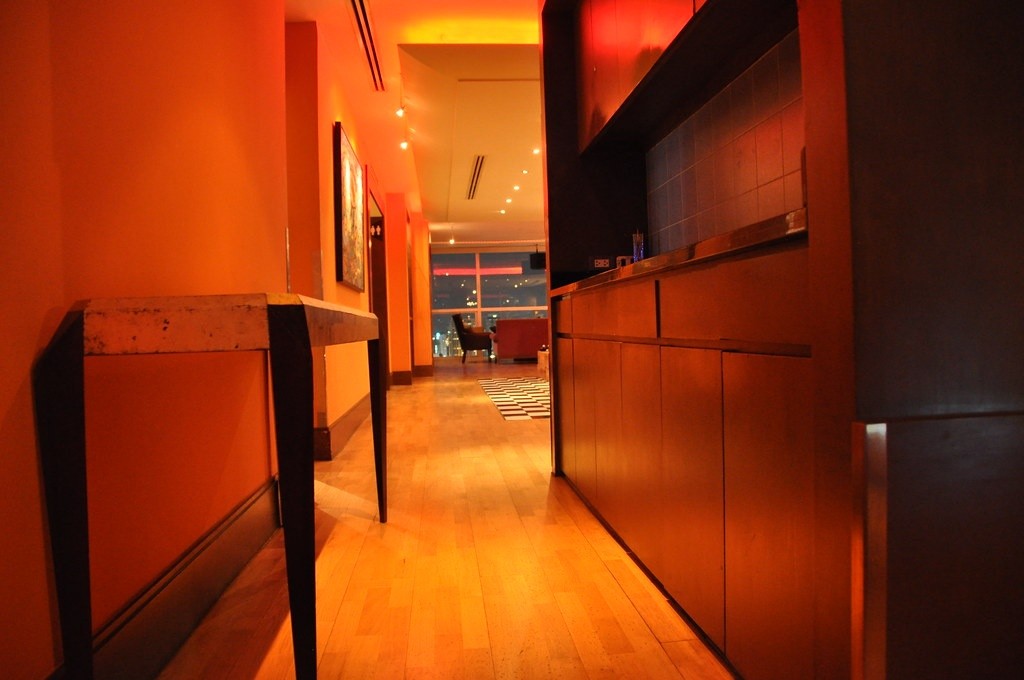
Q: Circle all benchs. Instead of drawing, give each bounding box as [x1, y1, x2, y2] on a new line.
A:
[493, 318, 548, 365]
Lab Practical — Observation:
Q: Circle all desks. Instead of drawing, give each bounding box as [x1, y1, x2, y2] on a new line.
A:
[30, 292, 388, 679]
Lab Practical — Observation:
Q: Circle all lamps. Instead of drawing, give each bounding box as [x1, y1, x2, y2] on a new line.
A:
[396, 103, 406, 117]
[400, 134, 414, 149]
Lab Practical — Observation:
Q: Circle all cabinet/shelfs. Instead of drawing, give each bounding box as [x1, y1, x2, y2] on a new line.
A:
[552, 234, 810, 680]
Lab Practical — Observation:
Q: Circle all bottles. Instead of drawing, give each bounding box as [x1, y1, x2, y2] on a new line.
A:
[633, 231, 644, 263]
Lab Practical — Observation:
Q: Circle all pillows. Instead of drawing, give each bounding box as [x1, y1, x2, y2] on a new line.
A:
[473, 327, 485, 333]
[464, 324, 473, 334]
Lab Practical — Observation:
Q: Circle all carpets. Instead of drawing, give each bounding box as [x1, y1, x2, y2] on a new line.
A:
[477, 377, 550, 420]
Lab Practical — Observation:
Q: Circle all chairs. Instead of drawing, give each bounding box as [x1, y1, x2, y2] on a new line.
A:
[452, 314, 492, 363]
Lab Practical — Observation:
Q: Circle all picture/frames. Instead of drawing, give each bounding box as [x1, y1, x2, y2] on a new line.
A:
[335, 122, 367, 291]
